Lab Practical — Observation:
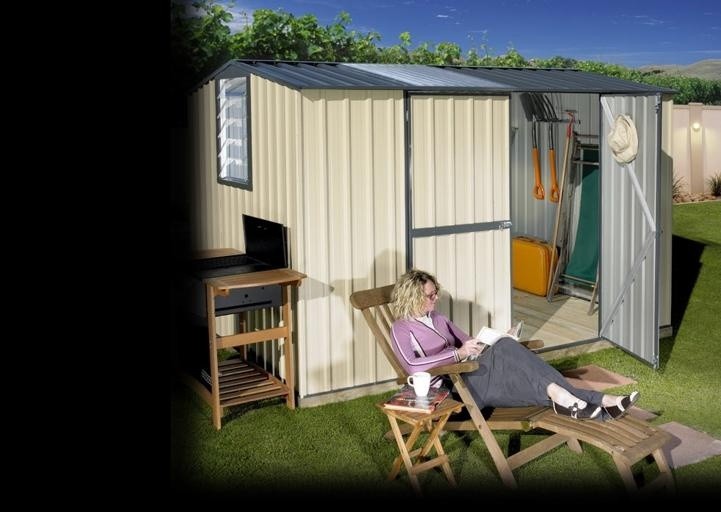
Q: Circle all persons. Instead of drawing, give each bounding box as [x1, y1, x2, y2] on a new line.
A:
[390, 269, 640, 420]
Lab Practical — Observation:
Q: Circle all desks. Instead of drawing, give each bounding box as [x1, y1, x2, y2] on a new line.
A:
[195, 247, 307, 431]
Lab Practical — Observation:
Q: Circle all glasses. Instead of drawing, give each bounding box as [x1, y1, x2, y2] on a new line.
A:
[427, 288, 438, 301]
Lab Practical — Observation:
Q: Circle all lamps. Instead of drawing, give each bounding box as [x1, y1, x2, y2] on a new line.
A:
[693, 122, 701, 131]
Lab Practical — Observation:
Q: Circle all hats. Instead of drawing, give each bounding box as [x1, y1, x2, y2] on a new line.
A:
[607, 113, 638, 164]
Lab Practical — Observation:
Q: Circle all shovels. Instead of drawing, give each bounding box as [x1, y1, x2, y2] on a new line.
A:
[519, 92, 546, 199]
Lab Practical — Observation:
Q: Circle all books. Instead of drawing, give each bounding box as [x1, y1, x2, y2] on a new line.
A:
[384, 384, 449, 414]
[461, 320, 524, 363]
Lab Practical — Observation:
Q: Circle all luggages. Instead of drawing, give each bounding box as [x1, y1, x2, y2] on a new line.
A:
[510, 236, 559, 297]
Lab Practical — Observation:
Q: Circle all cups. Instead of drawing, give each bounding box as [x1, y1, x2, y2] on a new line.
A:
[407, 372, 431, 397]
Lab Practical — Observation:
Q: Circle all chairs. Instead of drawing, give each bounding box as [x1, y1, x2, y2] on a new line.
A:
[349, 284, 675, 503]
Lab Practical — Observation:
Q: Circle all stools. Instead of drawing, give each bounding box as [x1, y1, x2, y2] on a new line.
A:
[375, 394, 465, 497]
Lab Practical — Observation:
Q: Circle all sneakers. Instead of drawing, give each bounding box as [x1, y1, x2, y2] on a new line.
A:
[552, 401, 601, 421]
[601, 391, 639, 422]
